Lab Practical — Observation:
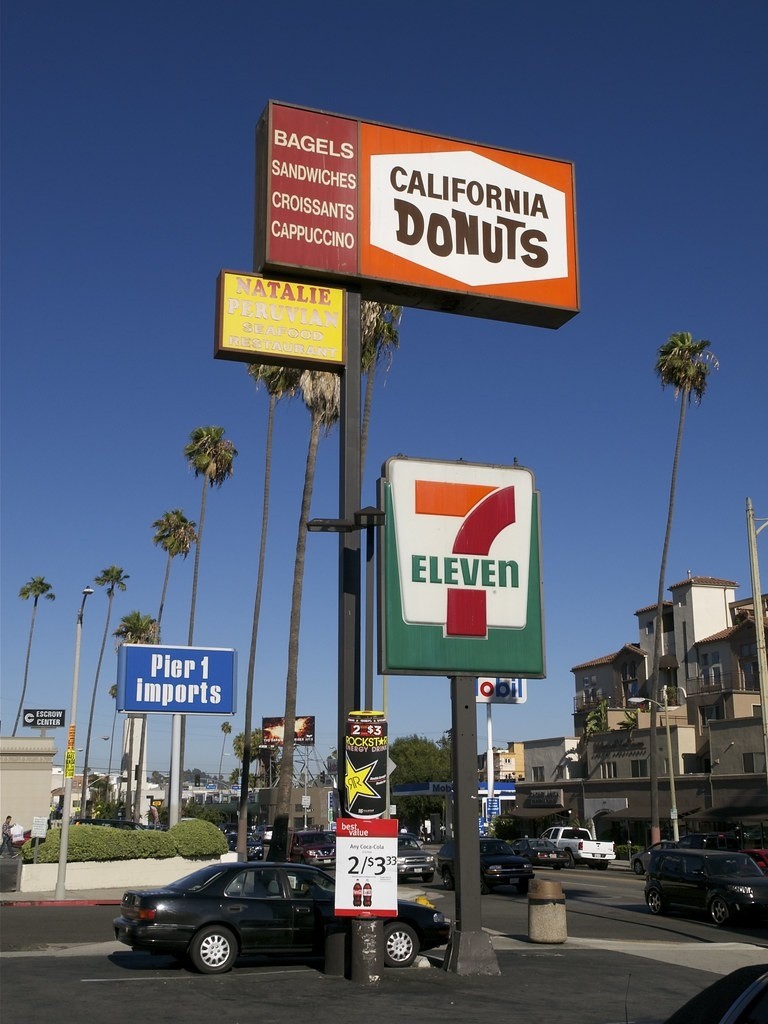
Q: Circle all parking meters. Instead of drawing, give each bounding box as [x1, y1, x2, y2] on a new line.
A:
[627, 840, 631, 861]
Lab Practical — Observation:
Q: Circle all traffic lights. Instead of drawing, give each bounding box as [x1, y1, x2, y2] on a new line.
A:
[195, 774, 200, 786]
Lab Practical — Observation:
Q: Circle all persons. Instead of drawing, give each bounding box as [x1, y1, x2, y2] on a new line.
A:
[0, 816, 18, 858]
[420, 824, 425, 842]
[401, 826, 407, 833]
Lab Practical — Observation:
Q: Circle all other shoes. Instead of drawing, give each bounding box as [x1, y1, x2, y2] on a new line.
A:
[0, 854, 5, 859]
[11, 853, 19, 858]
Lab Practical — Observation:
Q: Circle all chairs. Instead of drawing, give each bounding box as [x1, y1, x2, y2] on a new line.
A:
[263, 878, 281, 898]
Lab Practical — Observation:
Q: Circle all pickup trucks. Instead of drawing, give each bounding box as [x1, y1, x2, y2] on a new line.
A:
[537, 827, 616, 870]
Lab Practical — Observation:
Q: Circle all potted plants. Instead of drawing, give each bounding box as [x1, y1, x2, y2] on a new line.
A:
[665, 686, 677, 706]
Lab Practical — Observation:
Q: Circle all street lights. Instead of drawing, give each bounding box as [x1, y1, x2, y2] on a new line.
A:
[627, 696, 680, 844]
[54, 585, 95, 903]
[62, 736, 109, 786]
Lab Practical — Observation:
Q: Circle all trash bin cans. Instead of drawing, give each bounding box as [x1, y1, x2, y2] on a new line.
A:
[527, 878, 568, 944]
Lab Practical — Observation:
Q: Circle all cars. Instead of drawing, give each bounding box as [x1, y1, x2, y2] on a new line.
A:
[112, 861, 450, 974]
[11, 829, 31, 848]
[509, 839, 570, 870]
[398, 833, 424, 850]
[218, 824, 237, 835]
[630, 832, 768, 875]
[227, 834, 264, 860]
[143, 824, 168, 831]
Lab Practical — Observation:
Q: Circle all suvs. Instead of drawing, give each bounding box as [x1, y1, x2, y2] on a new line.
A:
[290, 831, 336, 867]
[436, 837, 535, 895]
[397, 835, 435, 883]
[643, 848, 768, 925]
[254, 825, 274, 843]
[70, 819, 145, 830]
